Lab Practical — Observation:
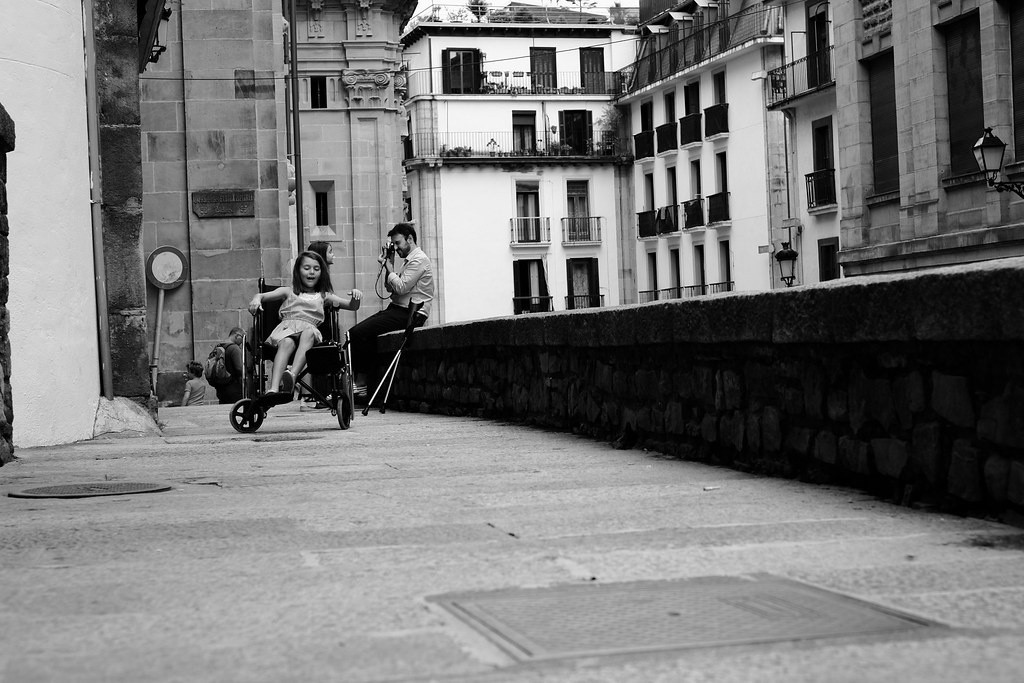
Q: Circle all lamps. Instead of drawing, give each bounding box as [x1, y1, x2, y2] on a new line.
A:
[972, 127, 1024, 197]
[774, 241, 798, 288]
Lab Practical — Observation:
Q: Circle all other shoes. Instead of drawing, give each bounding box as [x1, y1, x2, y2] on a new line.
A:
[357, 386, 367, 396]
[299, 400, 332, 412]
[265, 390, 277, 395]
[283, 370, 294, 394]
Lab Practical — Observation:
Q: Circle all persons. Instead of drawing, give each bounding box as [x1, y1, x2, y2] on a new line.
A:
[180, 361, 206, 407]
[299, 241, 334, 412]
[247, 250, 363, 395]
[345, 223, 435, 395]
[204, 327, 245, 404]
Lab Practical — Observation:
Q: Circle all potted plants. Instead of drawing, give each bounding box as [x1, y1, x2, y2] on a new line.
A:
[488, 138, 572, 157]
[586, 137, 605, 156]
[440, 144, 471, 157]
[615, 152, 635, 166]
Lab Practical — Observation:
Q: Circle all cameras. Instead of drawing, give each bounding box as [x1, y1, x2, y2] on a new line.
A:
[382, 242, 395, 259]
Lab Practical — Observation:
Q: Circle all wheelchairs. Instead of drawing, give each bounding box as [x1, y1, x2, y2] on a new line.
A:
[229, 277, 354, 433]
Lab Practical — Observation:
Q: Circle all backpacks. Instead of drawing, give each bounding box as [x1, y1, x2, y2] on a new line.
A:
[204, 342, 235, 386]
